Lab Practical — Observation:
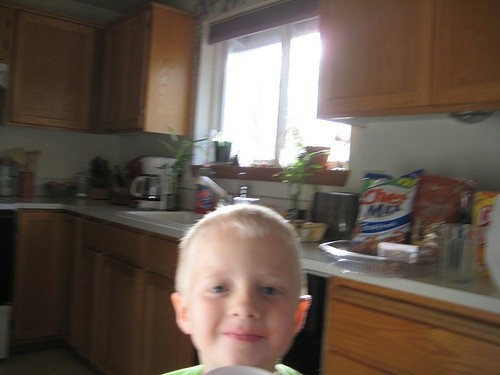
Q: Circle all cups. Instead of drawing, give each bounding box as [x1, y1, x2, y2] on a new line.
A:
[18, 172, 33, 197]
[436, 223, 481, 283]
[73, 167, 92, 197]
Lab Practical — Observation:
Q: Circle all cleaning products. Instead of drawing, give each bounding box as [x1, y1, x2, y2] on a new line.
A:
[194, 164, 215, 214]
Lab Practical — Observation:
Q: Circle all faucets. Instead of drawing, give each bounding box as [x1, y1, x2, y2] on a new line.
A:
[195, 175, 236, 205]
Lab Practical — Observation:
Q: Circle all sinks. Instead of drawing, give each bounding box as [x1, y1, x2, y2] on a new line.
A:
[117, 210, 209, 232]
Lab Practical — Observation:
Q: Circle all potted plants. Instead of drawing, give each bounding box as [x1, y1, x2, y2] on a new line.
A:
[90, 156, 113, 199]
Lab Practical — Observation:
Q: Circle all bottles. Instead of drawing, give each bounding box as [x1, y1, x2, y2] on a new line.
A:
[0, 159, 17, 197]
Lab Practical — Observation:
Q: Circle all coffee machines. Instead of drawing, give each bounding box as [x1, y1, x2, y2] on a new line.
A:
[129, 157, 181, 212]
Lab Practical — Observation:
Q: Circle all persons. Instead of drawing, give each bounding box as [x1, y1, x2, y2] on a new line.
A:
[164, 207, 313, 375]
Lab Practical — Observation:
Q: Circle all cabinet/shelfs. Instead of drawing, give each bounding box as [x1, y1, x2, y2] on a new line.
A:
[321, 279, 500, 375]
[317, 0, 500, 118]
[0, 0, 200, 137]
[12, 210, 194, 375]
